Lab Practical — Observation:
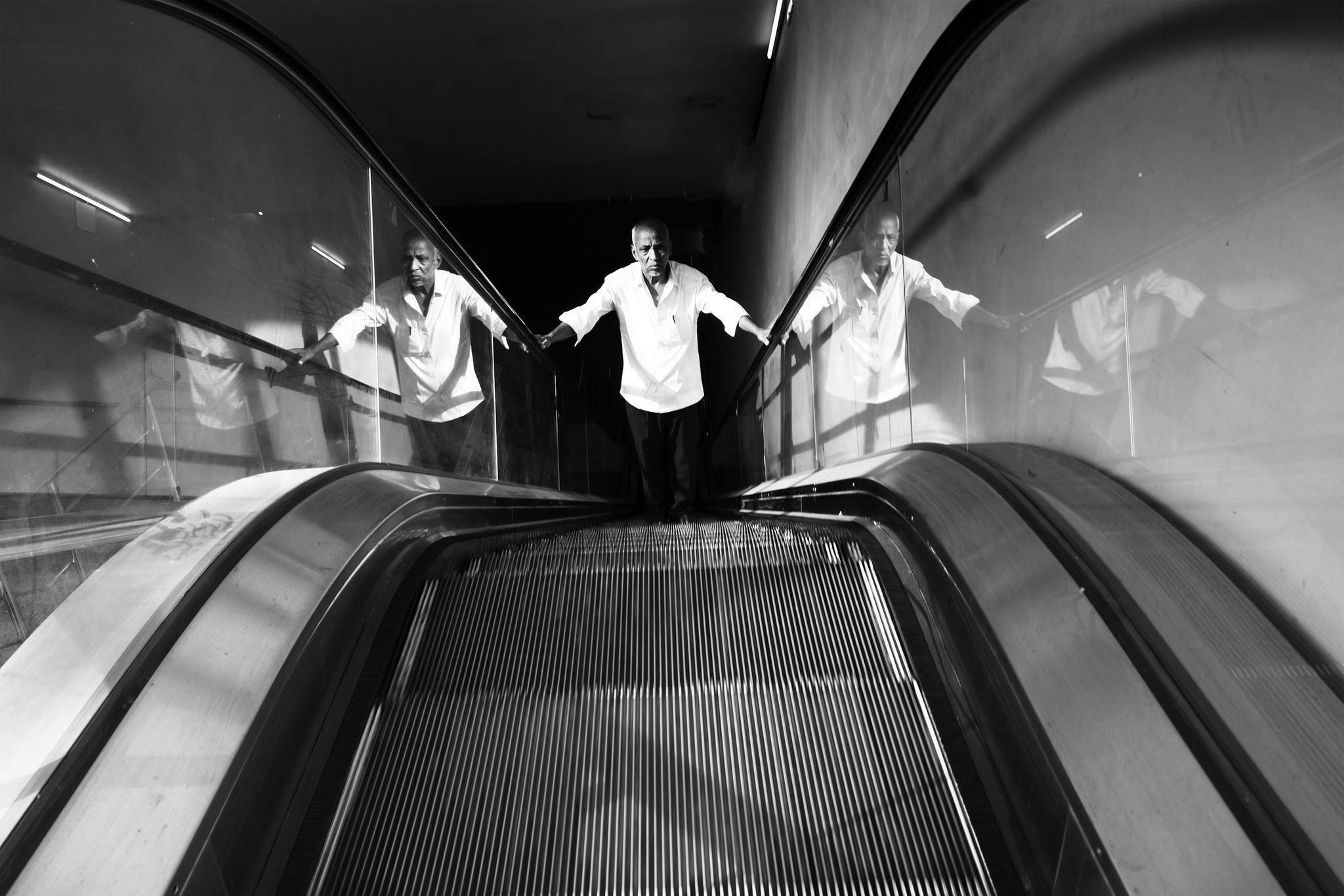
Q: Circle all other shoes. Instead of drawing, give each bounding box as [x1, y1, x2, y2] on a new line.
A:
[677, 514, 691, 524]
[651, 521, 664, 526]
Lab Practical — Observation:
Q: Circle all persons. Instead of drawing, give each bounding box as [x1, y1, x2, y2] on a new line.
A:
[287, 236, 520, 479]
[781, 207, 1031, 466]
[1039, 268, 1255, 456]
[520, 218, 772, 524]
[95, 309, 279, 470]
